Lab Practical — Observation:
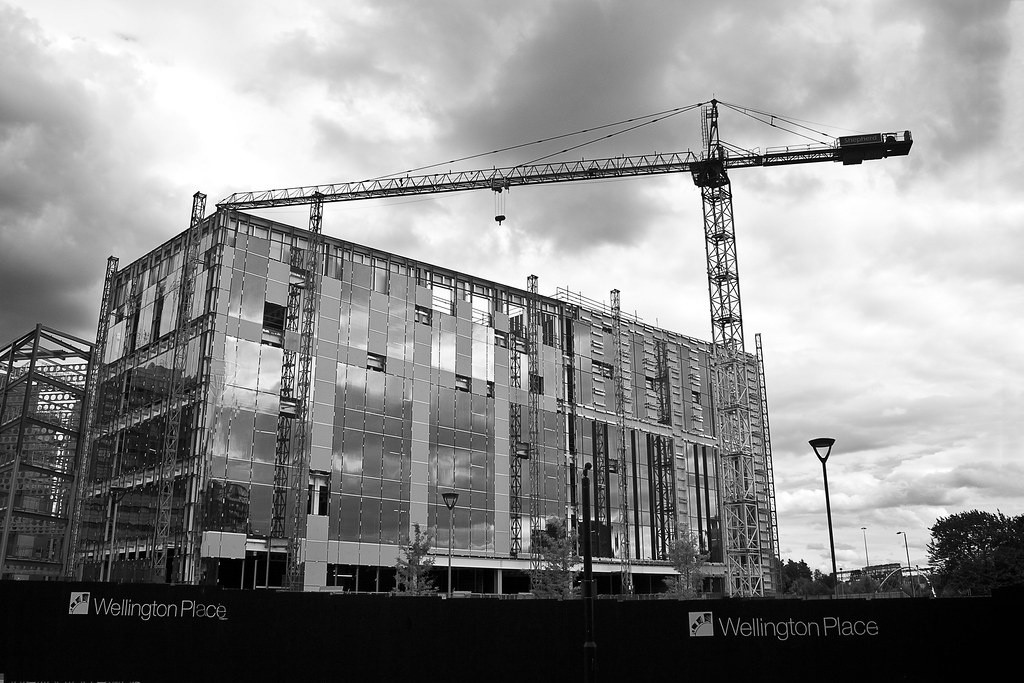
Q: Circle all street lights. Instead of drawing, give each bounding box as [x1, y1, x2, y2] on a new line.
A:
[897, 532, 913, 583]
[809, 437, 837, 579]
[441, 493, 460, 597]
[860, 527, 870, 566]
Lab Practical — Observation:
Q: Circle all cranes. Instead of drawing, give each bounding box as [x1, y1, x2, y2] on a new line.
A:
[215, 98, 914, 598]
